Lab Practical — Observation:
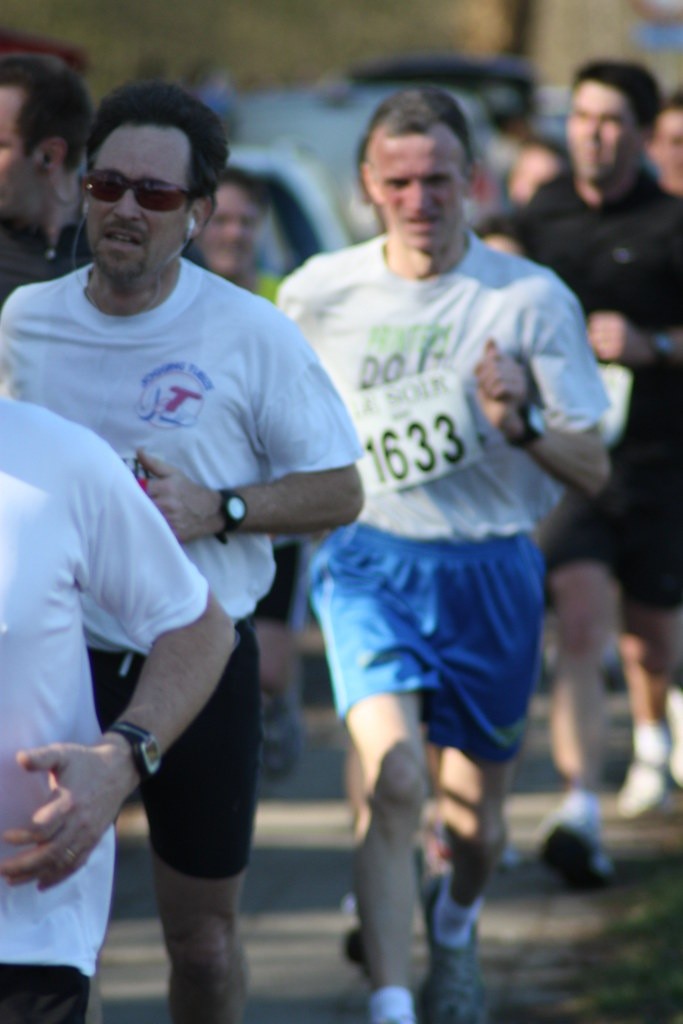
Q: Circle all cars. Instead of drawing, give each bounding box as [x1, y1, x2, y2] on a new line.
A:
[188, 50, 582, 297]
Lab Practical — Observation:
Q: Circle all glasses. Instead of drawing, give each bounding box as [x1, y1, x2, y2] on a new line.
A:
[82, 170, 192, 211]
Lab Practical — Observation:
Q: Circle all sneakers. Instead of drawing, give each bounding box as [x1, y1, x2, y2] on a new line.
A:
[415, 870, 489, 1024]
[537, 813, 615, 895]
[614, 763, 673, 821]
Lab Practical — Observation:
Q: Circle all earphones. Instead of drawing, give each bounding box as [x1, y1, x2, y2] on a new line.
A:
[187, 215, 197, 240]
[82, 195, 89, 213]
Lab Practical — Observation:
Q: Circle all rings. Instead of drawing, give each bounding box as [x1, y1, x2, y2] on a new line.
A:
[66, 849, 77, 859]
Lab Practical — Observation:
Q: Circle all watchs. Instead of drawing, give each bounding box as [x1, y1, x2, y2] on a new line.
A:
[112, 723, 161, 779]
[649, 331, 670, 361]
[218, 490, 247, 544]
[512, 402, 546, 445]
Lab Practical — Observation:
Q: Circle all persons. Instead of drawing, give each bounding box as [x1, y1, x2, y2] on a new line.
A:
[0, 58, 97, 310]
[0, 83, 364, 1024]
[273, 86, 611, 1024]
[193, 169, 310, 783]
[343, 54, 683, 965]
[0, 398, 236, 1024]
